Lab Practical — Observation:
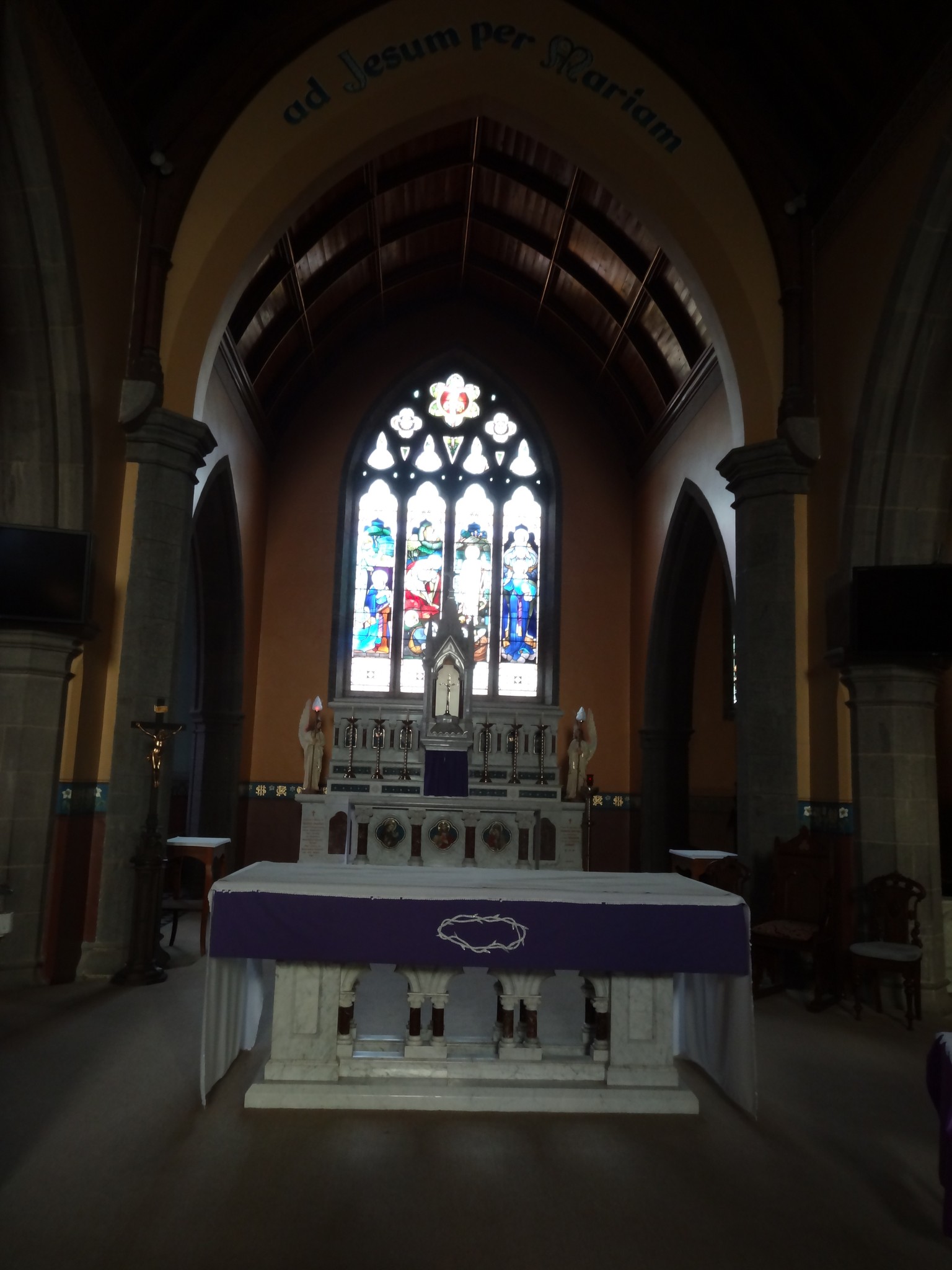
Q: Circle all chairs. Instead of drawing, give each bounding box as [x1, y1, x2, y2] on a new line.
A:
[749, 827, 841, 1011]
[850, 871, 927, 1030]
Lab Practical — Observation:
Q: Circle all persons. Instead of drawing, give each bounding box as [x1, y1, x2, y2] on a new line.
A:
[566, 729, 591, 798]
[304, 719, 325, 790]
[136, 722, 183, 788]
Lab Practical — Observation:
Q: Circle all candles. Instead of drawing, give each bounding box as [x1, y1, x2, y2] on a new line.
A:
[379, 707, 381, 718]
[515, 712, 518, 723]
[541, 712, 545, 725]
[407, 709, 409, 719]
[487, 710, 489, 721]
[352, 706, 354, 717]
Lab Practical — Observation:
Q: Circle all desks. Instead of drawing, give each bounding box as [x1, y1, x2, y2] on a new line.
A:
[670, 848, 737, 894]
[198, 861, 759, 1120]
[166, 836, 232, 956]
